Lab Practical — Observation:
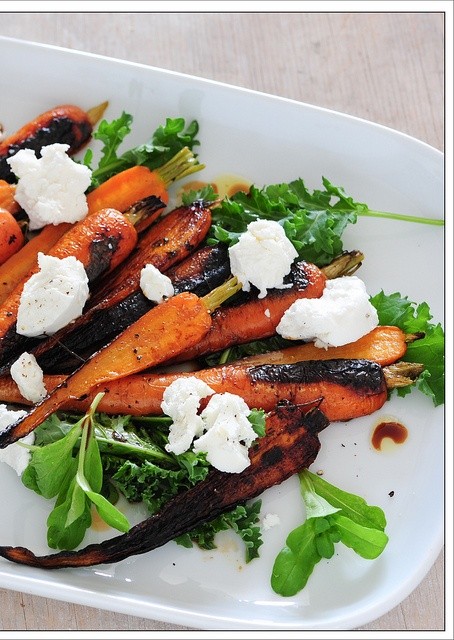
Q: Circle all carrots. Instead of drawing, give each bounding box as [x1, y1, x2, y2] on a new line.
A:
[0, 96, 424, 570]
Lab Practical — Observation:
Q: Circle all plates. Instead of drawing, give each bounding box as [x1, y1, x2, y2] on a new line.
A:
[0, 39, 444, 632]
